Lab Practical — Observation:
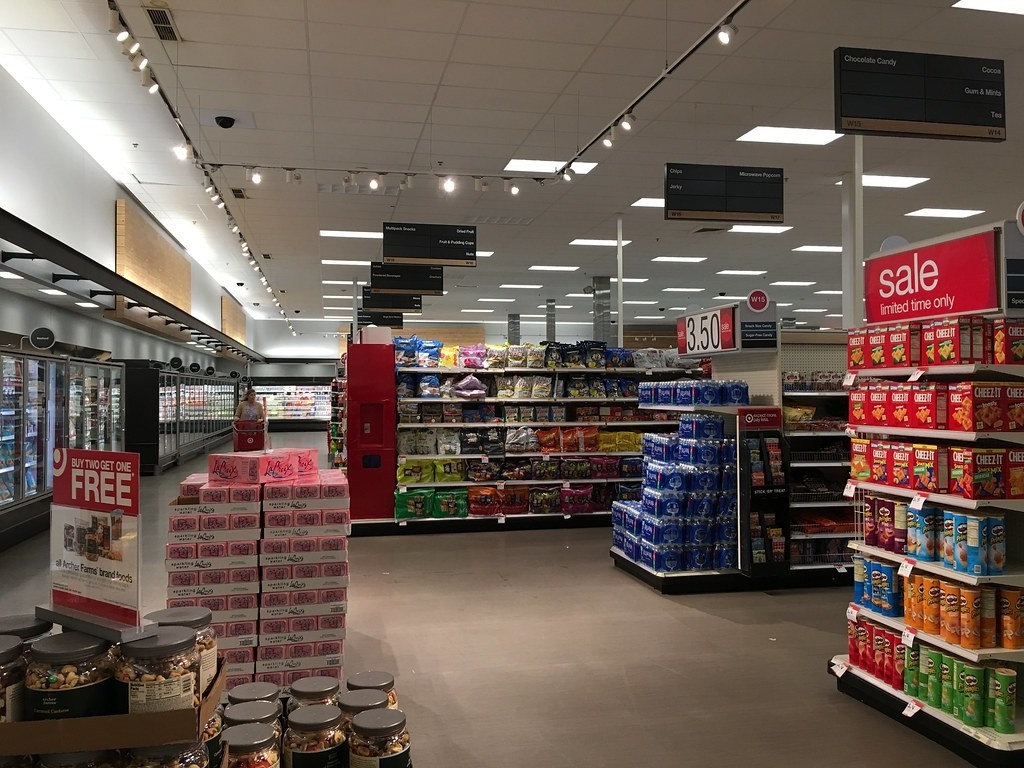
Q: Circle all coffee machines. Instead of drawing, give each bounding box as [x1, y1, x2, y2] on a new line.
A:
[642, 463, 716, 572]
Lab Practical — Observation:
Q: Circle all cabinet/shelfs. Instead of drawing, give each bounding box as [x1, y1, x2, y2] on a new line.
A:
[328, 301, 857, 595]
[825, 219, 1024, 768]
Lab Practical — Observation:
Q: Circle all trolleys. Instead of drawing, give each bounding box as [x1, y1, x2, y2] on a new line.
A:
[231, 418, 267, 452]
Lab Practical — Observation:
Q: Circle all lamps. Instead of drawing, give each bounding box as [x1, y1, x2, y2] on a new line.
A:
[718, 23, 738, 45]
[408, 175, 415, 189]
[439, 175, 446, 190]
[202, 175, 225, 208]
[474, 178, 481, 190]
[107, 10, 159, 94]
[226, 216, 295, 335]
[504, 180, 511, 192]
[351, 172, 359, 187]
[286, 171, 292, 182]
[622, 113, 637, 130]
[603, 126, 616, 148]
[563, 169, 575, 180]
[245, 168, 252, 180]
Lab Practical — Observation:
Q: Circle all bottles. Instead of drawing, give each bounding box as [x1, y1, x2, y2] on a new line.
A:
[719, 440, 738, 571]
[643, 433, 676, 462]
[638, 380, 750, 406]
[677, 441, 716, 463]
[613, 499, 642, 562]
[676, 413, 725, 440]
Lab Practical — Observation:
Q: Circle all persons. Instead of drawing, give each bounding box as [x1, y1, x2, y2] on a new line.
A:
[233, 389, 265, 423]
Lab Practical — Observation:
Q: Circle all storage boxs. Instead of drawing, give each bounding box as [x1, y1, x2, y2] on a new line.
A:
[0, 448, 351, 768]
[846, 313, 1024, 734]
